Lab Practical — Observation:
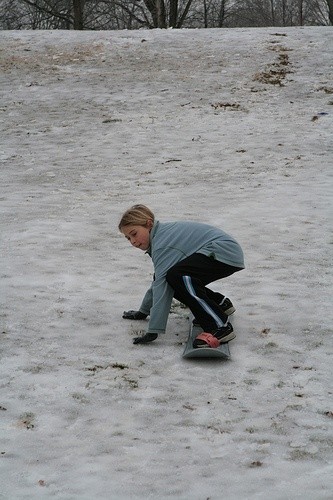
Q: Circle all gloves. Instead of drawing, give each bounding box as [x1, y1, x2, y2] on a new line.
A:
[133, 332, 158, 344]
[122, 310, 148, 319]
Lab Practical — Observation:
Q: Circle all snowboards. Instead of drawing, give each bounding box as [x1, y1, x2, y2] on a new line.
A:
[181, 321, 232, 359]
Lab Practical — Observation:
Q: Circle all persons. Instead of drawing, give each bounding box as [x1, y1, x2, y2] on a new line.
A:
[118, 204, 245, 348]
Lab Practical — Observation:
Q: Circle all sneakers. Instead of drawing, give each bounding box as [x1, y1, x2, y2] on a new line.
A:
[194, 322, 237, 348]
[193, 296, 236, 327]
